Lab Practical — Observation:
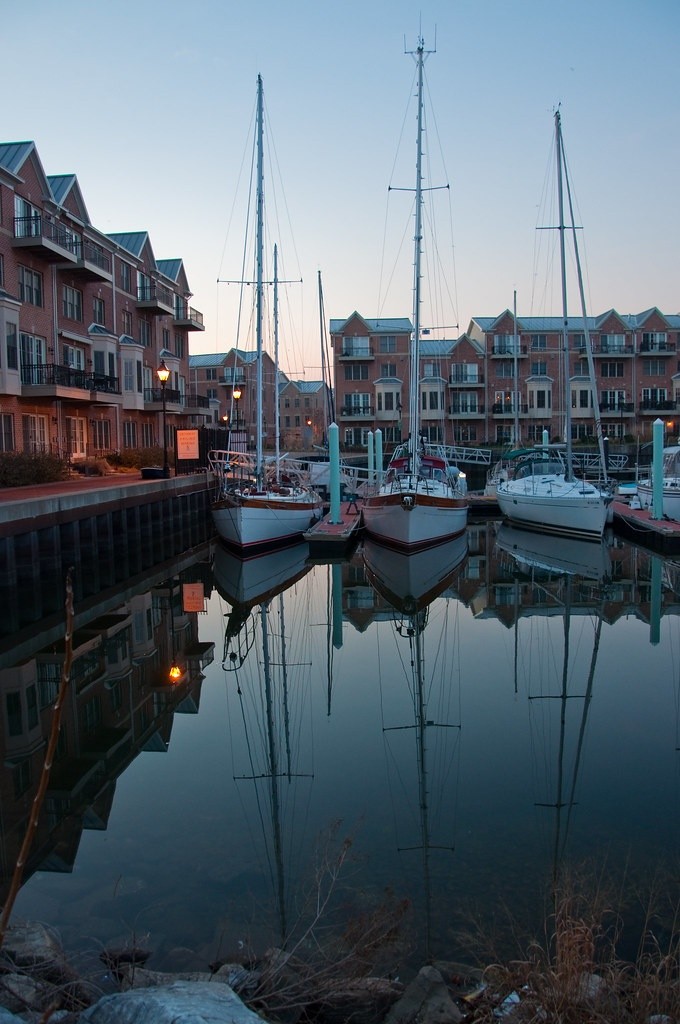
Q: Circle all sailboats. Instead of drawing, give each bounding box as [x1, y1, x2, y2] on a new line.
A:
[349, 28, 472, 542]
[488, 98, 611, 544]
[487, 522, 614, 890]
[208, 536, 312, 956]
[351, 537, 469, 954]
[211, 68, 325, 549]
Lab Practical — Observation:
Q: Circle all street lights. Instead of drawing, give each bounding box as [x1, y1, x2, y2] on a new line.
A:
[231, 383, 242, 432]
[156, 359, 171, 479]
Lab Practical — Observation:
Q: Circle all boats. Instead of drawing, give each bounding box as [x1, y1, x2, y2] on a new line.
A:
[637, 441, 680, 526]
